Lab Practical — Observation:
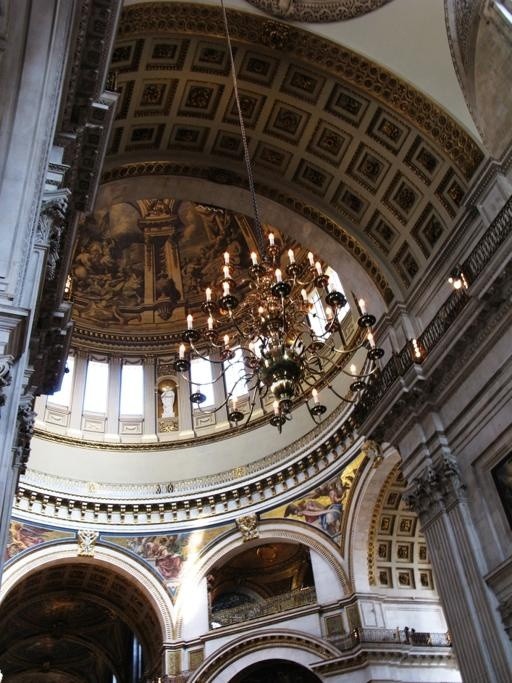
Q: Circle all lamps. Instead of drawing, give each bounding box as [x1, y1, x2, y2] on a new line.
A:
[448, 265, 469, 293]
[171, 2, 389, 437]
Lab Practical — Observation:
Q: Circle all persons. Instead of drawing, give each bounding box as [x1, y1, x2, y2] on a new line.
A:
[284, 476, 345, 542]
[161, 385, 175, 417]
[128, 535, 180, 579]
[7, 520, 42, 557]
[75, 196, 242, 319]
